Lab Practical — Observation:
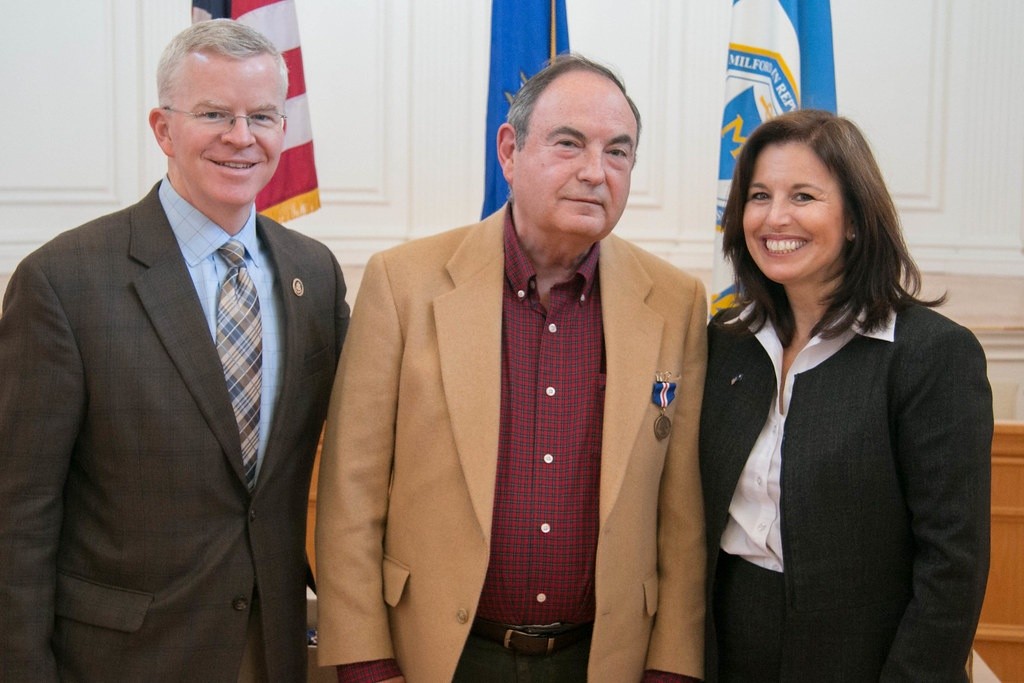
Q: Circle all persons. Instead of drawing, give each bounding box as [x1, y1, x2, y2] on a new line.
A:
[697, 111, 995, 683]
[314, 57, 709, 683]
[0, 18, 352, 682]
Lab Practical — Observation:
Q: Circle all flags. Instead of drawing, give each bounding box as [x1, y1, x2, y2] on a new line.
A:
[714, 0, 840, 243]
[191, 1, 323, 225]
[481, 0, 573, 219]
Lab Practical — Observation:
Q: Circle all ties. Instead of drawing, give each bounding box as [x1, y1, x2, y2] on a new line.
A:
[215, 238, 262, 498]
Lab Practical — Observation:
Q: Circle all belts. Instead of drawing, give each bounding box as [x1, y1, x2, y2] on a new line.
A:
[470, 624, 592, 653]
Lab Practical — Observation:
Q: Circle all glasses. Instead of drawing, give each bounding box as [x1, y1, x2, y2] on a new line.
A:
[162, 106, 287, 134]
